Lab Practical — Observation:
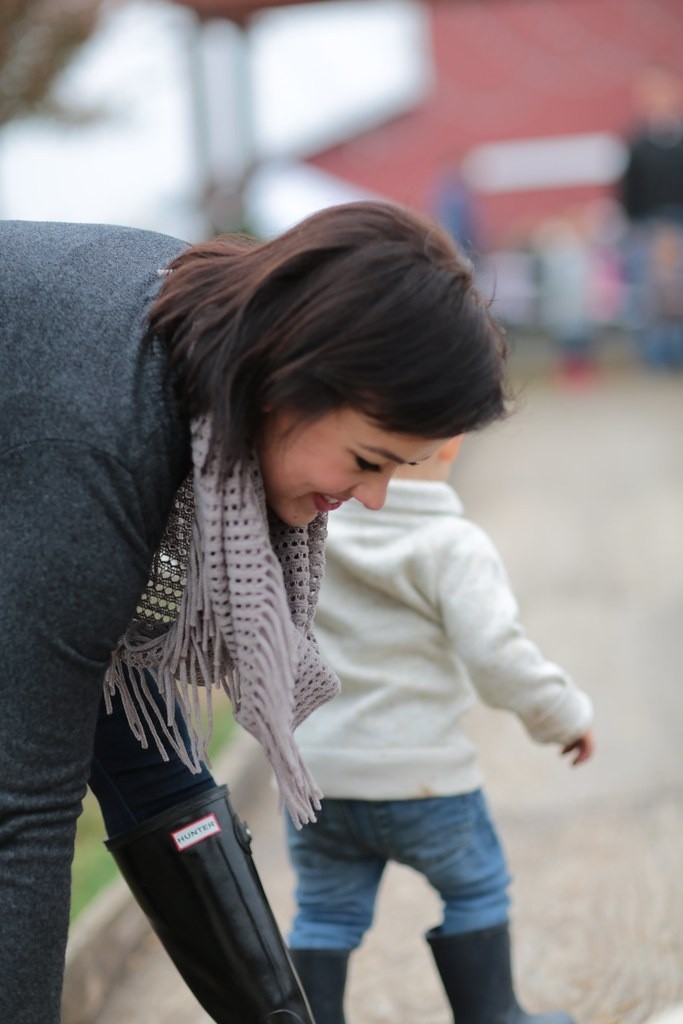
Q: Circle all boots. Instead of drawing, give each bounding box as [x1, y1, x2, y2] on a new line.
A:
[288, 947, 351, 1023]
[424, 922, 571, 1021]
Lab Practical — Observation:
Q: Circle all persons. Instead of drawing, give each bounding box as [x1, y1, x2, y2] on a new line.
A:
[0, 202, 518, 1023]
[277, 420, 603, 1024]
